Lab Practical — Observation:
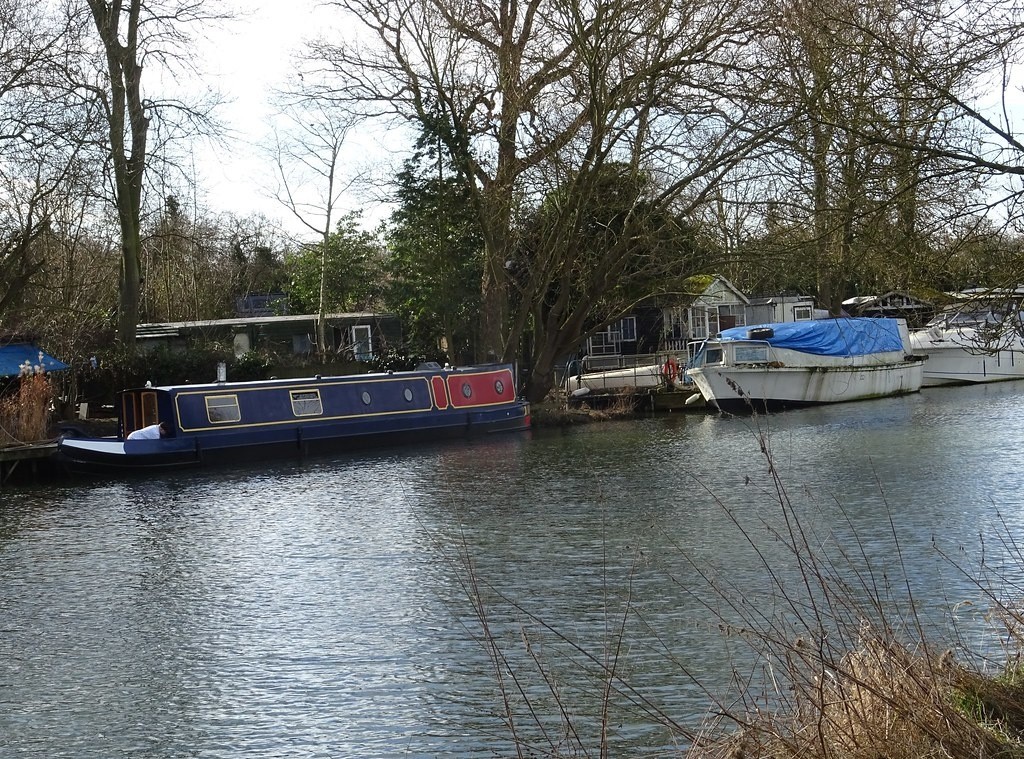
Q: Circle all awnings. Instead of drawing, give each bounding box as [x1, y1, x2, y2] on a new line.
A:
[0, 344, 71, 375]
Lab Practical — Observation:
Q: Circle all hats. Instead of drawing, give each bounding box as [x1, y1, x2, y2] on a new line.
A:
[160, 421, 170, 432]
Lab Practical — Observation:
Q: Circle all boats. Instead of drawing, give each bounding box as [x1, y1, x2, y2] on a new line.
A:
[58, 356, 533, 491]
[908, 285, 1024, 387]
[682, 309, 927, 413]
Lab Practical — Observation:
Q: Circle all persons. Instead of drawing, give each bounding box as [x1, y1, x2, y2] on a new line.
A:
[127, 421, 170, 439]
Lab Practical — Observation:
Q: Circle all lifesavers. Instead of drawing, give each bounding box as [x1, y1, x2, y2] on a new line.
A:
[664, 358, 678, 384]
